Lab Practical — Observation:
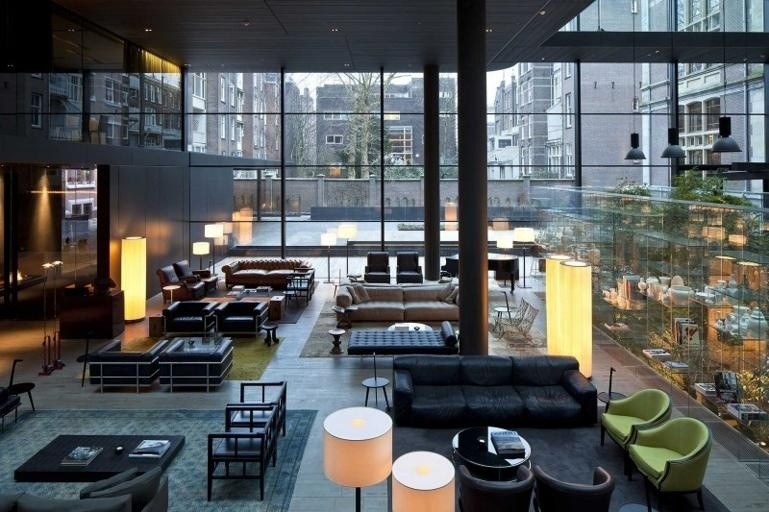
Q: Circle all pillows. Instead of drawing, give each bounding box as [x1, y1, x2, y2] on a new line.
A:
[72, 468, 140, 497]
[91, 463, 162, 506]
[438, 284, 459, 301]
[346, 284, 370, 303]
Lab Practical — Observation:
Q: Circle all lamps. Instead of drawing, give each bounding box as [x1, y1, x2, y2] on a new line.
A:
[202, 222, 225, 272]
[624, 131, 646, 165]
[38, 261, 55, 375]
[546, 253, 573, 355]
[711, 115, 741, 153]
[514, 227, 535, 288]
[190, 239, 213, 270]
[558, 260, 593, 379]
[661, 121, 687, 159]
[120, 235, 148, 323]
[48, 257, 64, 370]
[391, 449, 456, 512]
[321, 406, 392, 512]
[496, 235, 514, 254]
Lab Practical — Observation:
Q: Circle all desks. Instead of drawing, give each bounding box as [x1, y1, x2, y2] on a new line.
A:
[446, 247, 519, 289]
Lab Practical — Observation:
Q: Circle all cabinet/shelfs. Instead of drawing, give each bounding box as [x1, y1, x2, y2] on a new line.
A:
[56, 278, 126, 341]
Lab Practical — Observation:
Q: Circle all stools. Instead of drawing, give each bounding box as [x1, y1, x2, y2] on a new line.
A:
[7, 382, 37, 416]
[261, 322, 280, 345]
[346, 271, 362, 280]
[327, 327, 345, 354]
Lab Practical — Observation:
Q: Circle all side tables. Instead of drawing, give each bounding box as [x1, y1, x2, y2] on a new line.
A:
[359, 376, 392, 412]
[598, 389, 628, 409]
[494, 307, 512, 320]
[164, 284, 181, 304]
[270, 292, 286, 321]
[148, 311, 165, 337]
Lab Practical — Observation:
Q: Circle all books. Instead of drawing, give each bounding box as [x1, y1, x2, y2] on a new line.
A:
[642, 348, 688, 374]
[227, 285, 246, 298]
[491, 430, 526, 454]
[247, 285, 273, 294]
[695, 370, 768, 420]
[63, 447, 103, 466]
[129, 439, 170, 458]
[674, 316, 700, 347]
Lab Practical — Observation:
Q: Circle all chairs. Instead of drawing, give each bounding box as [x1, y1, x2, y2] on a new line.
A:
[498, 304, 539, 346]
[226, 381, 287, 440]
[287, 267, 316, 293]
[209, 405, 278, 501]
[283, 275, 312, 306]
[492, 297, 530, 333]
[0, 383, 23, 431]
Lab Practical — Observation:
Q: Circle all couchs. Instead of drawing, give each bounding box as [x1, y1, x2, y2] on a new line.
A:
[364, 252, 390, 283]
[392, 356, 600, 427]
[162, 300, 220, 336]
[396, 251, 423, 283]
[157, 337, 236, 387]
[600, 388, 673, 458]
[348, 320, 458, 356]
[88, 337, 171, 390]
[336, 282, 459, 326]
[531, 463, 617, 512]
[213, 301, 268, 338]
[0, 467, 168, 512]
[155, 265, 206, 299]
[457, 463, 534, 512]
[626, 418, 714, 511]
[174, 259, 218, 291]
[222, 256, 312, 290]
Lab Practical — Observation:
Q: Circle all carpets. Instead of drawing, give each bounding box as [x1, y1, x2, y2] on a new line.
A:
[0, 400, 318, 512]
[300, 294, 460, 360]
[384, 402, 732, 512]
[118, 328, 287, 381]
[206, 277, 319, 325]
